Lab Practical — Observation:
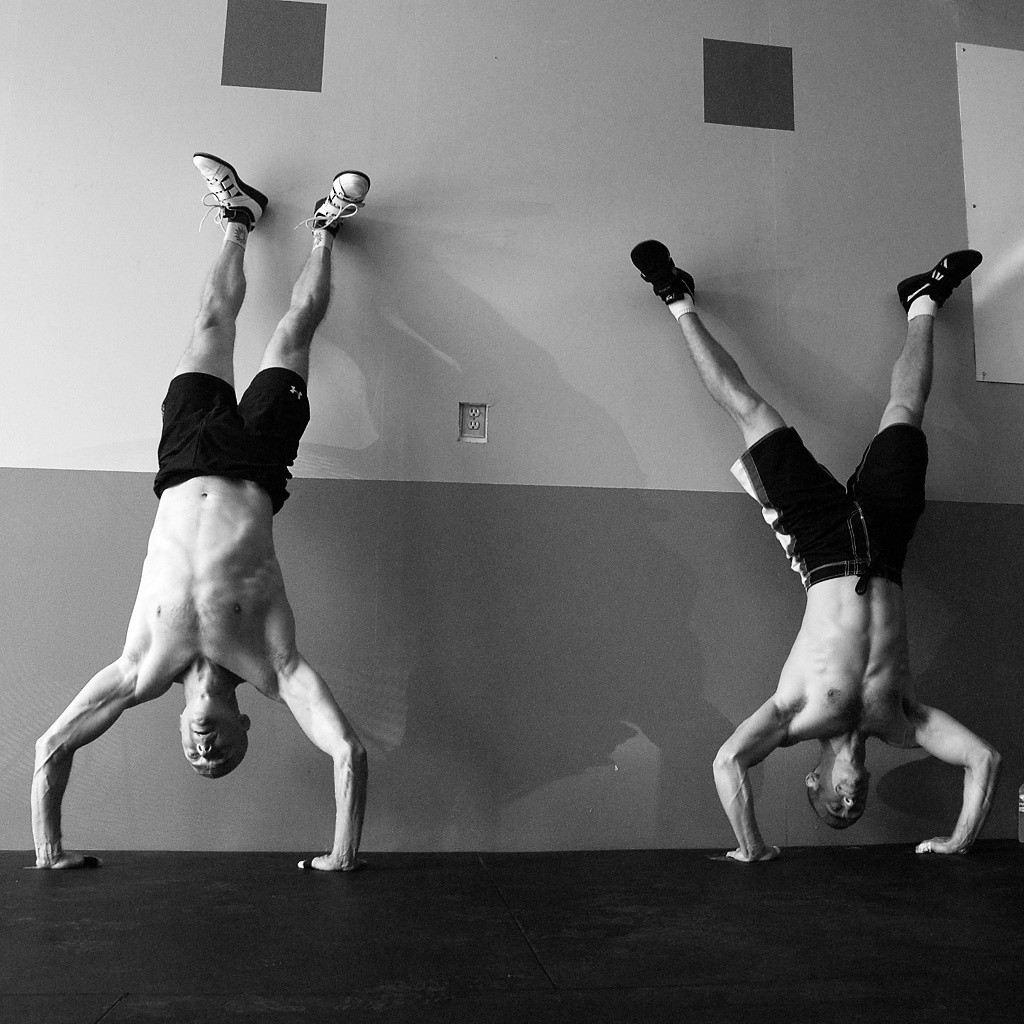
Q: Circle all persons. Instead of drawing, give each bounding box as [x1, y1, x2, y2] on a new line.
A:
[28, 152, 370, 876]
[627, 234, 1007, 859]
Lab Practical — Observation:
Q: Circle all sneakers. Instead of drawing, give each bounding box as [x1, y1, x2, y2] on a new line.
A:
[897, 250, 983, 317]
[192, 153, 269, 235]
[293, 171, 370, 237]
[630, 240, 694, 307]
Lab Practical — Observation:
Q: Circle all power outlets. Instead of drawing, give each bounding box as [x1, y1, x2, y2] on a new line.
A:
[461, 404, 486, 439]
